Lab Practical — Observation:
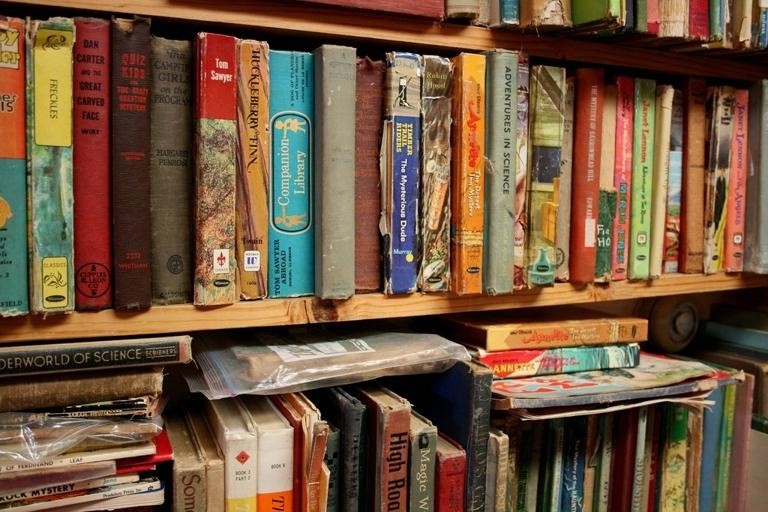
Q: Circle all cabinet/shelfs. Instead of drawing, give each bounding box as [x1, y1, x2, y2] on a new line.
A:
[0, 0, 766, 512]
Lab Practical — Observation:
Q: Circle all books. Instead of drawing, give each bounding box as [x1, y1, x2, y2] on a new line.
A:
[3, 13, 768, 321]
[395, 310, 767, 512]
[0, 332, 493, 512]
[443, 1, 767, 53]
